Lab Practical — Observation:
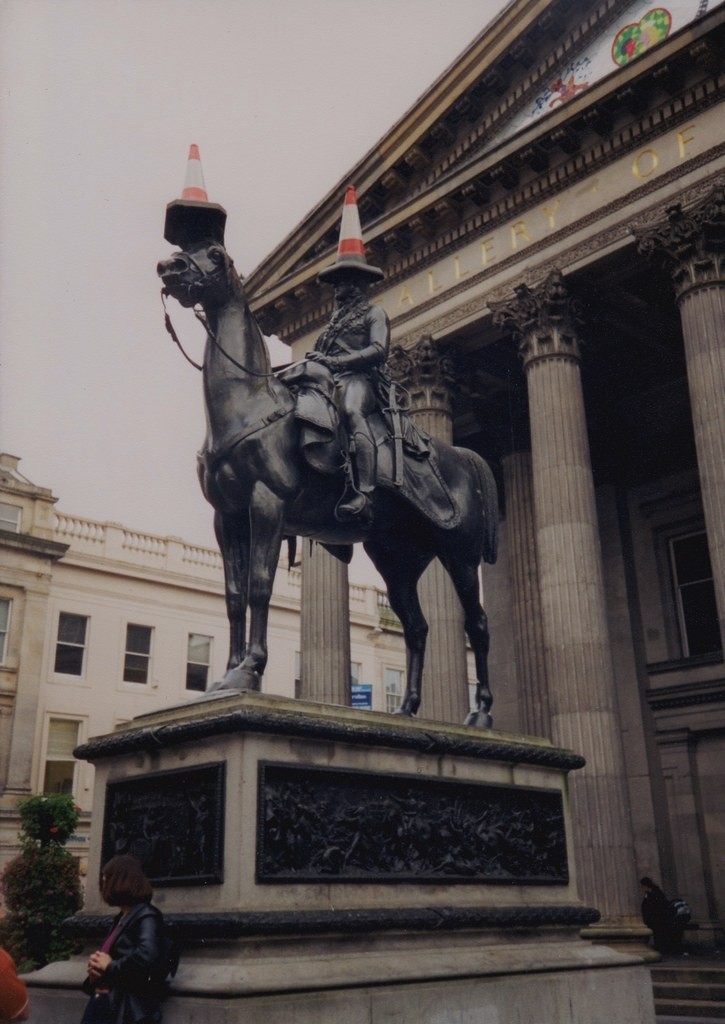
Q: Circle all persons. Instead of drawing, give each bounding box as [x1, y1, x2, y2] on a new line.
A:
[0, 947, 31, 1024]
[81, 854, 168, 1024]
[640, 877, 691, 959]
[309, 268, 392, 521]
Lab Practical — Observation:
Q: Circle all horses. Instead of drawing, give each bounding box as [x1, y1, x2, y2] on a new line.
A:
[157, 234, 499, 728]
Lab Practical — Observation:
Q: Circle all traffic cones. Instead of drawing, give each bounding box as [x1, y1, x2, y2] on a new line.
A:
[161, 142, 227, 245]
[316, 184, 385, 287]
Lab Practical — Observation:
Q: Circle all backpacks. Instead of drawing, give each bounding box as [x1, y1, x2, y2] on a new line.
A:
[139, 916, 178, 981]
